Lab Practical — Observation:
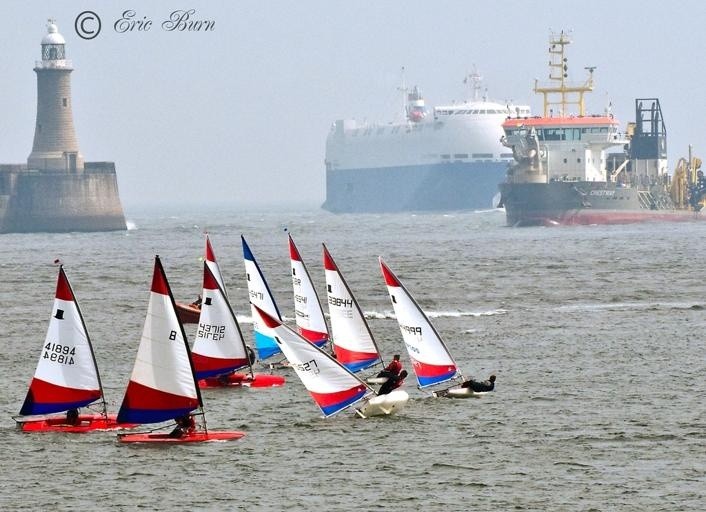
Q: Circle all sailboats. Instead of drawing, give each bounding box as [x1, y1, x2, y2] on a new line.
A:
[10, 229, 493, 447]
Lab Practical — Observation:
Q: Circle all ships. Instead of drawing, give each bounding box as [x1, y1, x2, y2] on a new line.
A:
[496, 27, 706, 227]
[321, 61, 532, 216]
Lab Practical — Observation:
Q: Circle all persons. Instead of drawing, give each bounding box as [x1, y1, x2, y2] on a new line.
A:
[188, 294, 201, 310]
[688, 170, 706, 211]
[460, 374, 496, 393]
[375, 369, 408, 396]
[166, 415, 195, 439]
[376, 354, 401, 378]
